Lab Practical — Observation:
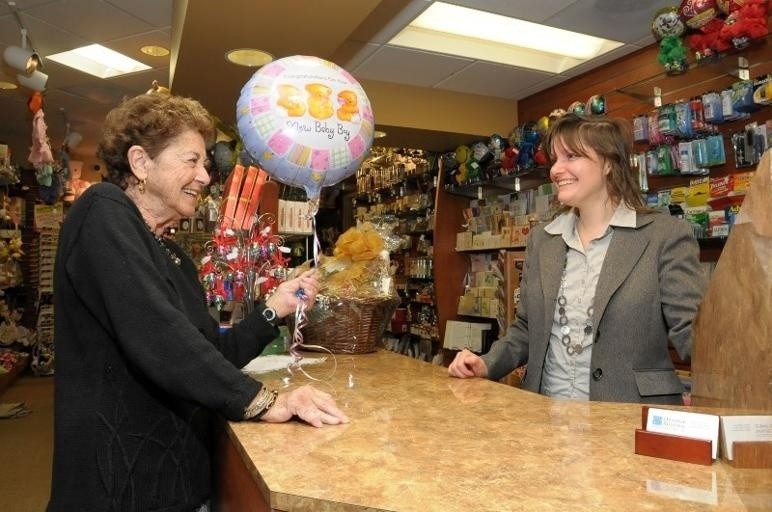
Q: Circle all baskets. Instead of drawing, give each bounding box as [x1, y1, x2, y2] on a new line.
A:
[284, 295, 401, 355]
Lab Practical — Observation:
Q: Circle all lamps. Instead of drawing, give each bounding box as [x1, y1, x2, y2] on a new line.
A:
[3, 27, 52, 93]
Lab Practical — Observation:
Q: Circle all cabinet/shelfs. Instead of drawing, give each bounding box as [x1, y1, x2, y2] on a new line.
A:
[342, 146, 576, 386]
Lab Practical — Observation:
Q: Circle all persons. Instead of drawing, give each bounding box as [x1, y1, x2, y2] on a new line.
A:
[447, 113, 704, 405]
[46, 90, 350, 511]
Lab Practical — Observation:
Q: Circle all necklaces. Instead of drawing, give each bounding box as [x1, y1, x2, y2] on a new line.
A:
[143, 219, 181, 265]
[556, 247, 594, 355]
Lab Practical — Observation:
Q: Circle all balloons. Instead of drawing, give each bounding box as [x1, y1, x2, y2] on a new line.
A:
[236, 55, 375, 199]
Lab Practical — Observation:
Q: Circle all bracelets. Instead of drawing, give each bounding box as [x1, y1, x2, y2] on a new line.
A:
[258, 301, 279, 324]
[243, 386, 279, 420]
